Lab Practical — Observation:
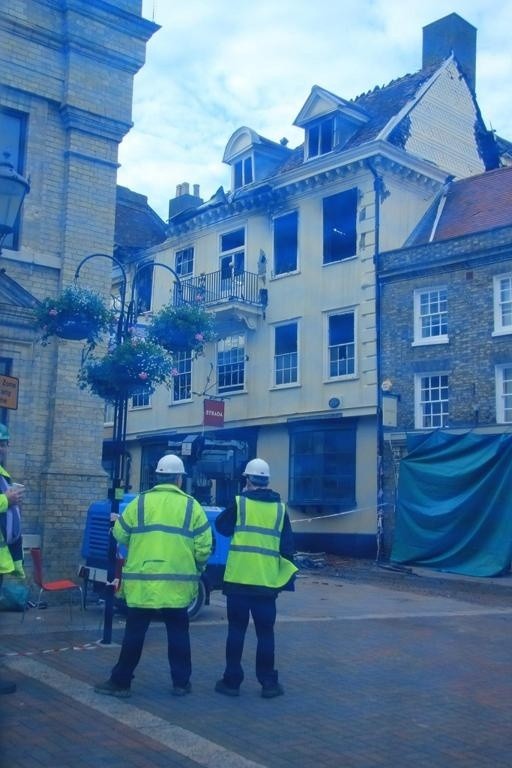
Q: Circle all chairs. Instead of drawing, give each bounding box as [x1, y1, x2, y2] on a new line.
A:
[19, 544, 88, 631]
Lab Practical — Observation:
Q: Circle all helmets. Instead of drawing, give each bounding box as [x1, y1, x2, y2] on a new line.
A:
[242, 458, 271, 478]
[0, 423, 8, 441]
[155, 454, 187, 475]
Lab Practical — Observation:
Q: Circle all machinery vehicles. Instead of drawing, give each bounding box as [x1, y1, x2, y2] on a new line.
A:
[76, 492, 232, 624]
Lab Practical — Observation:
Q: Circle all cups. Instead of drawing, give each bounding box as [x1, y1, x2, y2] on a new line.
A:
[12, 482, 25, 491]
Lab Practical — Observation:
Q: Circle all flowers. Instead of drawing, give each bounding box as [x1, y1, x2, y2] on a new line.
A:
[33, 279, 220, 409]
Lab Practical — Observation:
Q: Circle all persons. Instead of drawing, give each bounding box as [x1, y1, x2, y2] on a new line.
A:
[0, 424, 27, 695]
[93, 454, 212, 695]
[214, 458, 296, 699]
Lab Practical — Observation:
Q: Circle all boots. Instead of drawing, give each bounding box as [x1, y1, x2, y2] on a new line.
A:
[1, 681, 15, 694]
[261, 684, 283, 698]
[171, 681, 192, 695]
[214, 680, 240, 696]
[94, 677, 132, 698]
